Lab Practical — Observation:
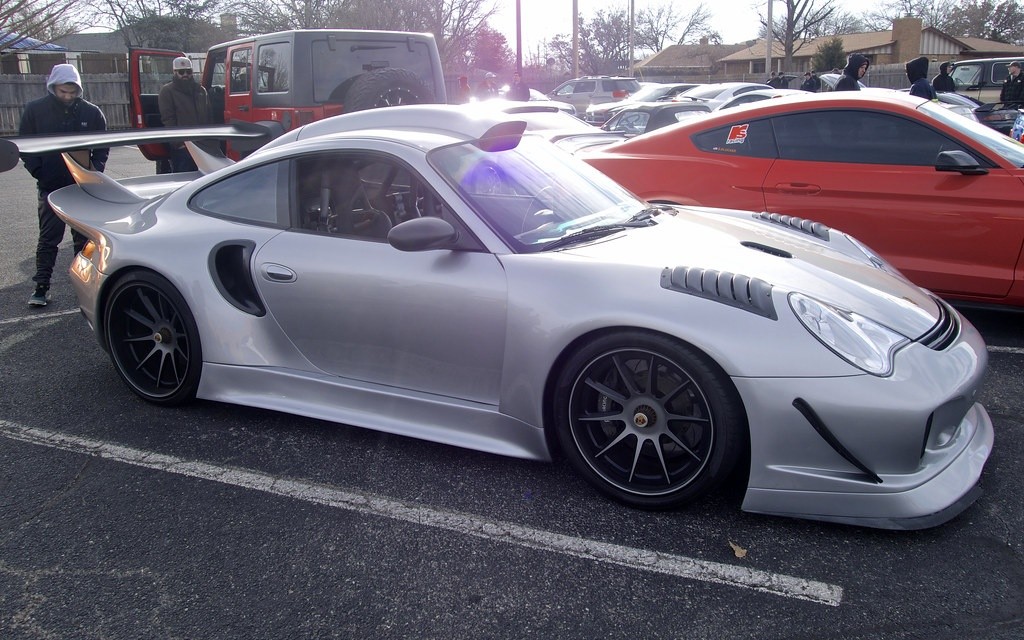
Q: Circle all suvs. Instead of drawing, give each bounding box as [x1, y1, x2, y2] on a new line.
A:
[546, 75, 641, 118]
[127, 29, 447, 174]
[930, 56, 1024, 106]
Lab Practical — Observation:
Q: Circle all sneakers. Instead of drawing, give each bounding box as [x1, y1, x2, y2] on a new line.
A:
[27, 284, 52, 306]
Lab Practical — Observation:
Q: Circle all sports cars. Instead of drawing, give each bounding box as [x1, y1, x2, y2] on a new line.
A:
[0, 98, 993, 531]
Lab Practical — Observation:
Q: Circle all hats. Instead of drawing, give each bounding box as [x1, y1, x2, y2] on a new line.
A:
[1005, 61, 1021, 68]
[173, 57, 193, 70]
[831, 68, 838, 72]
[803, 72, 810, 76]
[947, 61, 954, 67]
[457, 75, 467, 80]
[484, 72, 496, 78]
[858, 59, 868, 68]
[777, 72, 784, 76]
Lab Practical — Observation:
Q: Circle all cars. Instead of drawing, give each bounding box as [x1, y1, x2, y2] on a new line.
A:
[577, 90, 1024, 321]
[496, 88, 577, 118]
[587, 83, 866, 135]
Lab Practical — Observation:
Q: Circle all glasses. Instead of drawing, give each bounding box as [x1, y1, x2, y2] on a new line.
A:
[514, 74, 518, 76]
[174, 69, 193, 75]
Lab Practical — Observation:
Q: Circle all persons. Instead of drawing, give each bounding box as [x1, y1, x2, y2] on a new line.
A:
[932, 61, 956, 92]
[1000, 61, 1024, 109]
[800, 70, 821, 93]
[905, 56, 938, 101]
[825, 68, 841, 92]
[506, 71, 530, 102]
[833, 54, 870, 91]
[766, 71, 788, 89]
[16, 64, 110, 308]
[476, 71, 498, 102]
[157, 57, 216, 173]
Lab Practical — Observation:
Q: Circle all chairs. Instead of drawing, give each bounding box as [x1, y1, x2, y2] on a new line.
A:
[317, 159, 392, 242]
